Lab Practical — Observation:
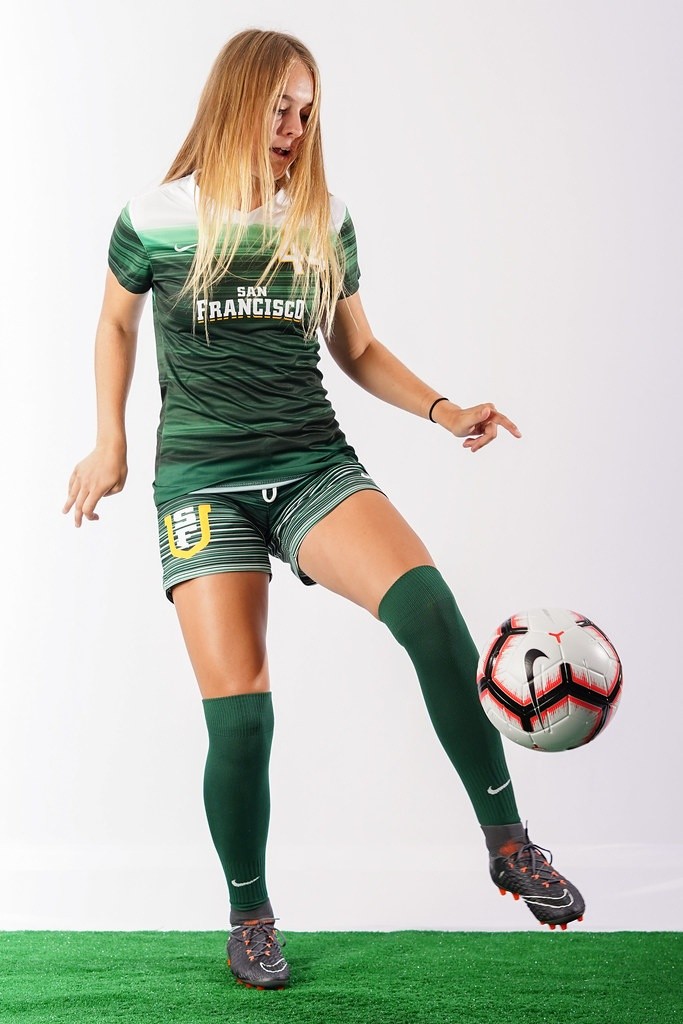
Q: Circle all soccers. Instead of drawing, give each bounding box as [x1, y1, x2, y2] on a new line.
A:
[476, 609, 623, 751]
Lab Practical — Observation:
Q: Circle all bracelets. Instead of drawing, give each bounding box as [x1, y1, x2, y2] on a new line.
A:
[429, 397, 449, 423]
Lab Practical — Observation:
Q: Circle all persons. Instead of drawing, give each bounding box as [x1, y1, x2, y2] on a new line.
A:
[63, 27, 585, 990]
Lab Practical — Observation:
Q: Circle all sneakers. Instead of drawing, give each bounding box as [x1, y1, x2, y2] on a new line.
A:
[488, 838, 586, 931]
[225, 915, 292, 992]
